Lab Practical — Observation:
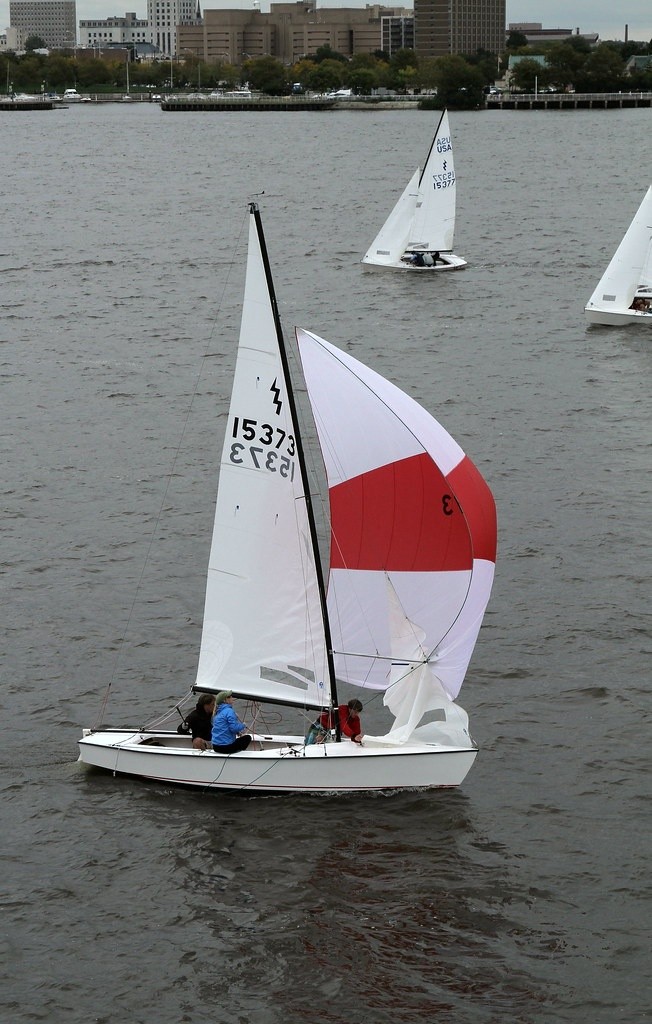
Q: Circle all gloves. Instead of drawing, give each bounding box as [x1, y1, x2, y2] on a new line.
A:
[238, 731, 242, 736]
[243, 722, 247, 727]
[182, 723, 188, 729]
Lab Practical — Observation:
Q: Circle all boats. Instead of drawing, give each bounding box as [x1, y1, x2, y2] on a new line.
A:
[152, 94, 162, 102]
[0, 88, 90, 106]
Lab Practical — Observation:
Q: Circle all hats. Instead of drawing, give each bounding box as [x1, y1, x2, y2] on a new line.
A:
[216, 690, 232, 704]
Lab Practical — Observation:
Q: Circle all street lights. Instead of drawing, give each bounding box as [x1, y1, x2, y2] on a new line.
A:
[65, 30, 77, 89]
[185, 47, 201, 89]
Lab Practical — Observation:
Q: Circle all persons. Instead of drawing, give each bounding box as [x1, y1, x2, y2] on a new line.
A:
[211, 690, 251, 754]
[177, 694, 216, 751]
[304, 699, 363, 746]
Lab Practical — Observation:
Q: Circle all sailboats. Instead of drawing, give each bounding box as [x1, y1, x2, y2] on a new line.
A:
[361, 107, 471, 271]
[75, 204, 501, 798]
[584, 180, 652, 329]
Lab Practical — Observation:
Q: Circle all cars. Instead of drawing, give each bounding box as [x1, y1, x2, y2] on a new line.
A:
[490, 86, 504, 95]
